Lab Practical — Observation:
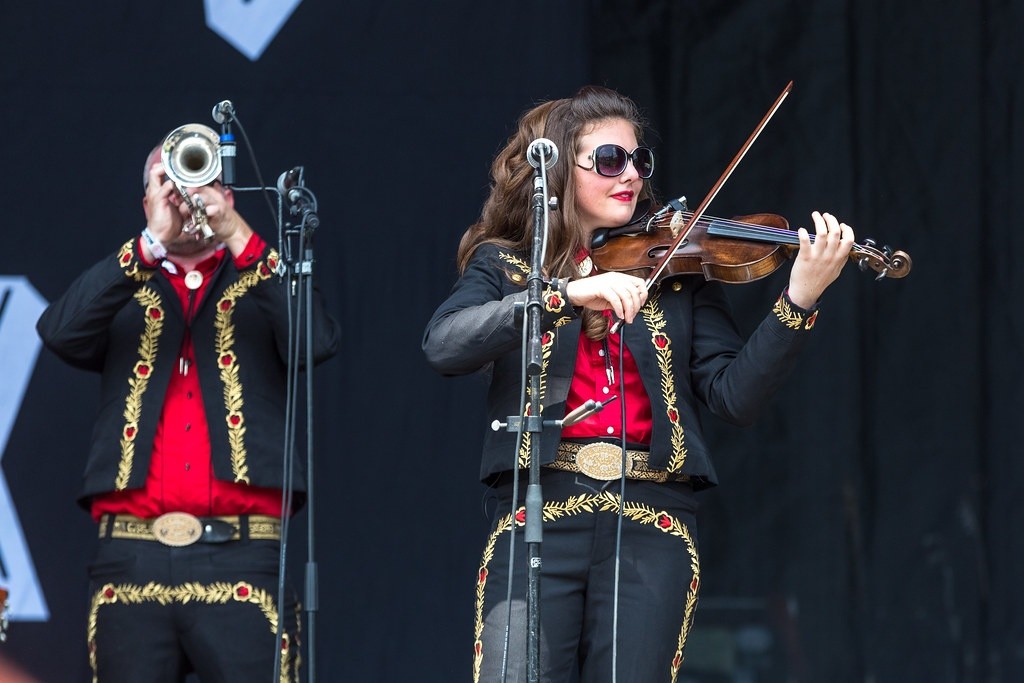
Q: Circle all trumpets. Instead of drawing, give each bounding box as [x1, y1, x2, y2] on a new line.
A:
[160, 122, 223, 245]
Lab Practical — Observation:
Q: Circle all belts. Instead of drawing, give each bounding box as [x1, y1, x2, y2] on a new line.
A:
[99, 512, 281, 547]
[542, 442, 690, 483]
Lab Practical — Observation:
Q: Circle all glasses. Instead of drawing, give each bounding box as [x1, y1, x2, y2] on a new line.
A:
[574, 144, 655, 179]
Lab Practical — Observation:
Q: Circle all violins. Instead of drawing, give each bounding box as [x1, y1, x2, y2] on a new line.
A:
[591, 203, 913, 293]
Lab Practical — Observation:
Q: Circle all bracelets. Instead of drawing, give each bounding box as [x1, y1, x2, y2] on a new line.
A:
[140, 228, 167, 259]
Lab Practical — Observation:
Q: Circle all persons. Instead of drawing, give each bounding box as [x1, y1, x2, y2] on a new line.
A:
[421, 87, 856, 683]
[37, 137, 342, 683]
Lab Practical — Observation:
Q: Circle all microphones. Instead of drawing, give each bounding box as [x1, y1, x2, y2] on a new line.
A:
[212, 100, 235, 124]
[527, 138, 558, 170]
[277, 166, 305, 196]
